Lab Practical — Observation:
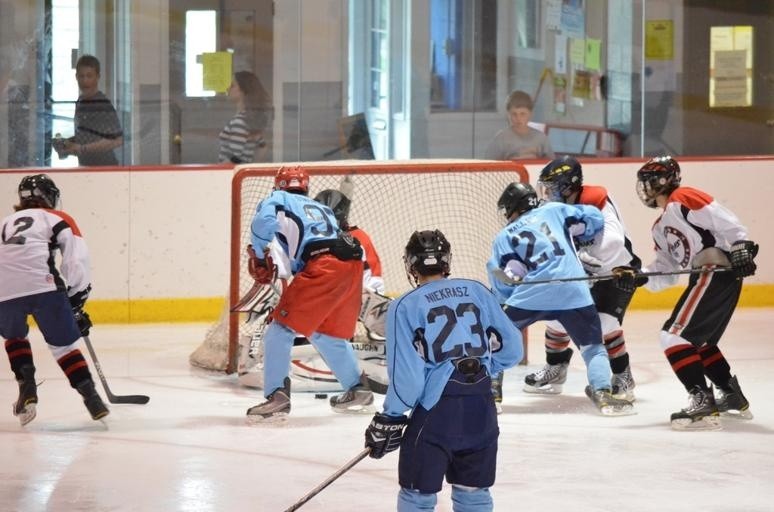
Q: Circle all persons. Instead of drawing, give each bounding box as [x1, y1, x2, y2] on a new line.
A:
[52, 55, 123, 166]
[0, 174, 110, 425]
[483, 90, 555, 160]
[216, 71, 274, 164]
[312, 189, 384, 295]
[486, 182, 633, 415]
[364, 228, 524, 512]
[635, 155, 758, 431]
[246, 165, 373, 423]
[522, 155, 647, 395]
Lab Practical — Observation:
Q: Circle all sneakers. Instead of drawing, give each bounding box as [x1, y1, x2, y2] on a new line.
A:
[247, 377, 289, 416]
[490, 378, 502, 403]
[12, 365, 37, 414]
[607, 371, 635, 394]
[670, 388, 718, 420]
[524, 363, 572, 386]
[77, 378, 108, 420]
[593, 390, 632, 409]
[714, 378, 749, 411]
[330, 379, 373, 407]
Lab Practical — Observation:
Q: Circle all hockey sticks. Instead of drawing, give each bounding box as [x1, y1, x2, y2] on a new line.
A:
[248, 244, 389, 395]
[493, 267, 733, 286]
[74, 310, 149, 404]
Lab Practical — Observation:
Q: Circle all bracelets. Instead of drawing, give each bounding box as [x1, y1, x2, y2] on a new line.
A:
[80, 144, 87, 153]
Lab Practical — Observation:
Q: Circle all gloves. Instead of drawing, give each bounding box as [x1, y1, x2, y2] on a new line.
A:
[364, 412, 406, 460]
[612, 265, 648, 293]
[246, 245, 273, 283]
[730, 239, 757, 280]
[74, 311, 92, 337]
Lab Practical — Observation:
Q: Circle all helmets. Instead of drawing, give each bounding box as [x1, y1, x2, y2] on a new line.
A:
[636, 156, 680, 191]
[313, 190, 350, 222]
[18, 174, 59, 208]
[496, 182, 536, 216]
[536, 155, 582, 188]
[274, 165, 308, 195]
[402, 229, 451, 275]
